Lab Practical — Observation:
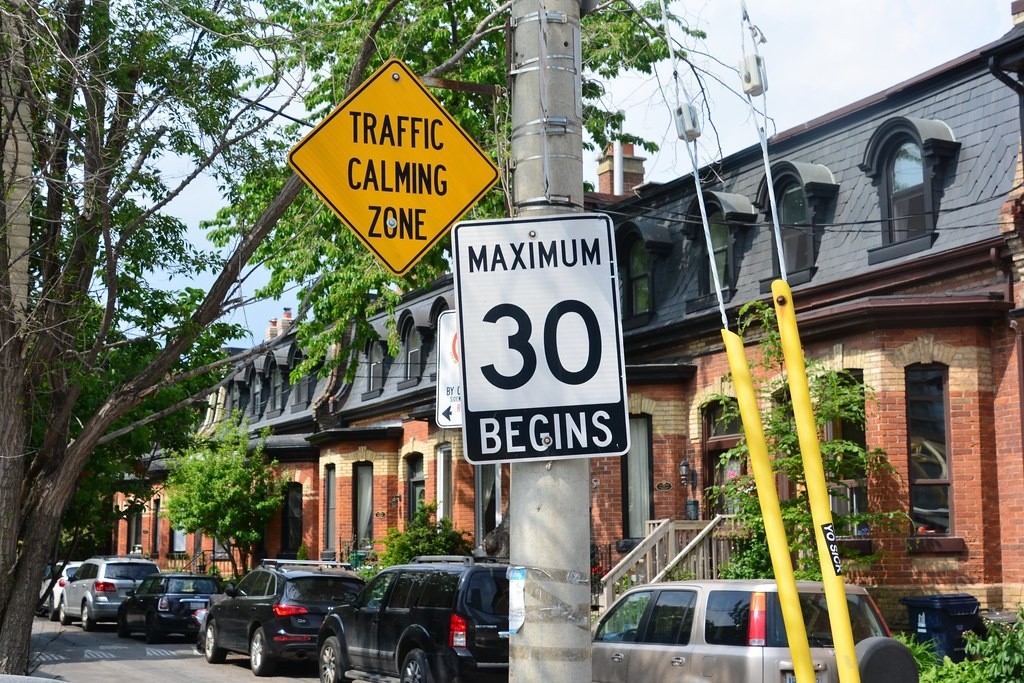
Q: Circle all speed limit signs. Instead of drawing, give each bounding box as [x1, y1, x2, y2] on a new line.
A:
[452, 215, 632, 465]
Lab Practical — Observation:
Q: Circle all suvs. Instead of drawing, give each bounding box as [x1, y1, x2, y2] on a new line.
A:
[316, 554, 509, 683]
[35, 555, 162, 633]
[196, 558, 367, 677]
[590, 579, 920, 683]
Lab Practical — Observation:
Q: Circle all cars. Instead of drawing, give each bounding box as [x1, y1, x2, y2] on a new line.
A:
[115, 572, 225, 644]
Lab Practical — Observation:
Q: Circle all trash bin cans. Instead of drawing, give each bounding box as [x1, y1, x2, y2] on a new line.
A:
[899, 592, 981, 666]
[349, 550, 377, 571]
[975, 607, 1018, 641]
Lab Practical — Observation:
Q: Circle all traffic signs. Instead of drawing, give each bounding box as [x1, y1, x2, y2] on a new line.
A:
[434, 307, 465, 430]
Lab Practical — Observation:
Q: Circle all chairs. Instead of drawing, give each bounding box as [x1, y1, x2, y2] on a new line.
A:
[173, 582, 184, 592]
[192, 582, 203, 593]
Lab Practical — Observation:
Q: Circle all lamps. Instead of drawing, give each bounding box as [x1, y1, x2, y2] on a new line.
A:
[679, 458, 697, 489]
[391, 494, 401, 508]
